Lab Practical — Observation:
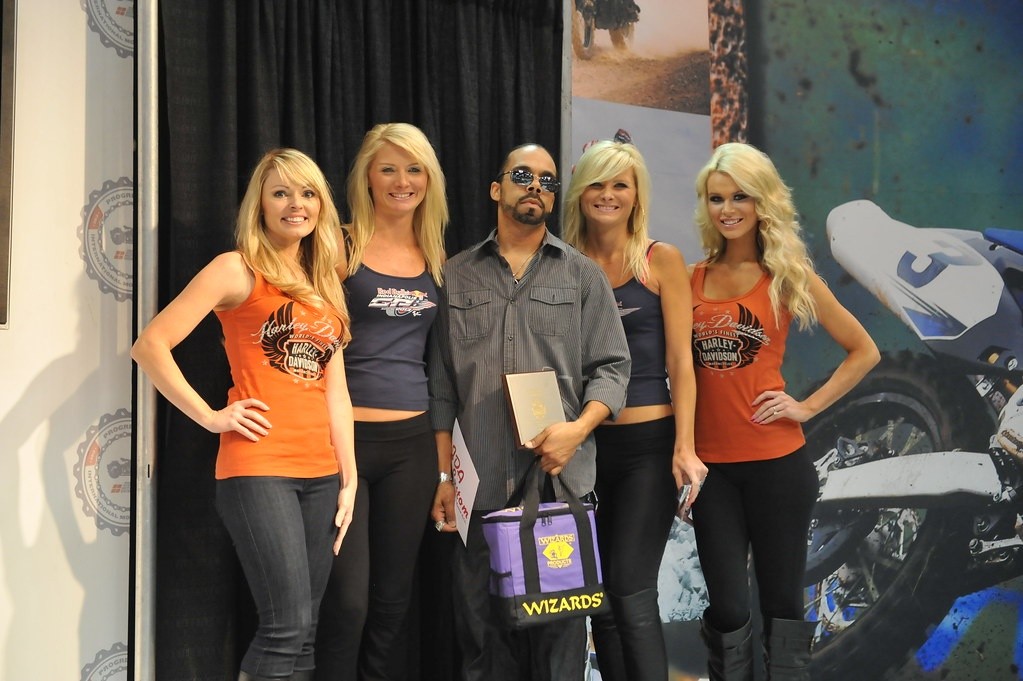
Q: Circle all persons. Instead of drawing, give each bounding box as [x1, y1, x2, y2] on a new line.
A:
[130, 150, 357, 681]
[313, 122, 446, 681]
[426, 144, 631, 681]
[677, 143, 880, 681]
[562, 142, 708, 681]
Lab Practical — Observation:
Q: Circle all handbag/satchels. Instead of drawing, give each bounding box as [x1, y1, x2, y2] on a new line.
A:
[480, 454, 611, 631]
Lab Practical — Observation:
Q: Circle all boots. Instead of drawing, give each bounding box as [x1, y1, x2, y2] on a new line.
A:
[590, 581, 627, 681]
[607, 587, 669, 681]
[698, 607, 754, 681]
[760, 615, 822, 681]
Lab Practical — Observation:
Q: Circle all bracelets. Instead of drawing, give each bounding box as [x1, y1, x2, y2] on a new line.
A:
[438, 472, 452, 483]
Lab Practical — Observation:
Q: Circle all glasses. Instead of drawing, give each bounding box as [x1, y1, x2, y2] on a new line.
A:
[496, 169, 561, 192]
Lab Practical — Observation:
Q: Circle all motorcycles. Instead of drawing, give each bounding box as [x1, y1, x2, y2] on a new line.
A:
[568, 1, 641, 60]
[750, 197, 1021, 681]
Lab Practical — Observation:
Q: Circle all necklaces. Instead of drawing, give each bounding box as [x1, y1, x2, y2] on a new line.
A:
[514, 245, 543, 276]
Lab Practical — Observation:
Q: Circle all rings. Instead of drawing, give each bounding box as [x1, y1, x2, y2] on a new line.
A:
[435, 521, 446, 532]
[699, 481, 703, 488]
[773, 406, 776, 413]
